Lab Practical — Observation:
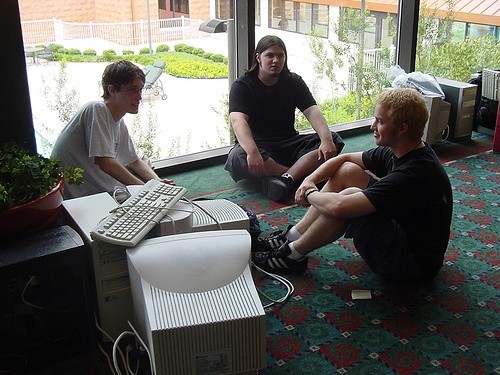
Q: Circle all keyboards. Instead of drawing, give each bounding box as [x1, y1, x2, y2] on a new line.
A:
[90, 179, 188, 247]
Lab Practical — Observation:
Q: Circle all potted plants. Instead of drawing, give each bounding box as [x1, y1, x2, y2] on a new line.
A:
[0, 139, 85, 240]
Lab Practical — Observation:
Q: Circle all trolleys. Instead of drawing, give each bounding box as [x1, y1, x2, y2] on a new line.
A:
[142, 59, 169, 100]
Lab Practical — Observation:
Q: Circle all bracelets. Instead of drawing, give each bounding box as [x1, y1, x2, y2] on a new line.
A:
[305, 189, 318, 201]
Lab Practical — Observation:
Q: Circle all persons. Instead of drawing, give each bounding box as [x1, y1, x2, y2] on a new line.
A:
[251, 89, 453, 281]
[50, 61, 174, 199]
[225, 36, 345, 202]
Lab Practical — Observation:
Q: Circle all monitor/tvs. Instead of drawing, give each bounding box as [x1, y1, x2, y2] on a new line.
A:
[125, 183, 251, 238]
[124, 228, 267, 375]
[419, 95, 451, 151]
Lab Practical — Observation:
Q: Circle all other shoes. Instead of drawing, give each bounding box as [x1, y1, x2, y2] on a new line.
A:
[259, 176, 296, 202]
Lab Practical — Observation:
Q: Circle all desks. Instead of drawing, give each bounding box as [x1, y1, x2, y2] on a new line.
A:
[23, 47, 44, 66]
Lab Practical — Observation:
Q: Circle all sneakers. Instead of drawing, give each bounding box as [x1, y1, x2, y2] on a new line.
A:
[251, 223, 295, 252]
[250, 239, 308, 278]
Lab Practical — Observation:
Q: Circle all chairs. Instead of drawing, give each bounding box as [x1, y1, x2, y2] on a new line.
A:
[36, 46, 55, 65]
[143, 60, 167, 105]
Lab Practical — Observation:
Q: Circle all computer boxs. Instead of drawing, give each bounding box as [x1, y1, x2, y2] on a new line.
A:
[434, 76, 478, 144]
[60, 192, 143, 342]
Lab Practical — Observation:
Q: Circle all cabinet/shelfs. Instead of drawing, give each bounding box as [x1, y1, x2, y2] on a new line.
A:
[0, 225, 92, 375]
